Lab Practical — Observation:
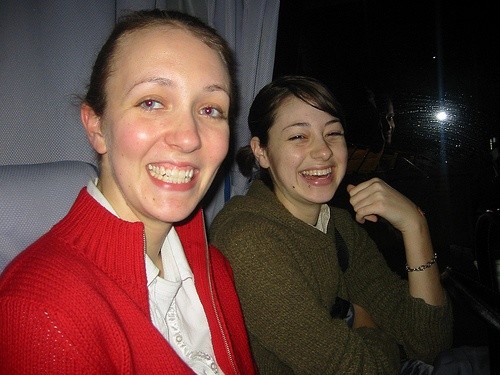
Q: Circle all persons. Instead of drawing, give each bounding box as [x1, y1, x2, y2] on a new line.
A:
[0, 9, 258, 375]
[339, 96, 399, 186]
[207, 75, 490, 375]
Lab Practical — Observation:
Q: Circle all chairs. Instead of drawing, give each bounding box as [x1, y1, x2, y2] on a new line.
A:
[0, 160, 98, 275]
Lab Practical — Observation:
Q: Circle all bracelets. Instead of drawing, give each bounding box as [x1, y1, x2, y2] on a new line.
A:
[405, 251, 438, 272]
